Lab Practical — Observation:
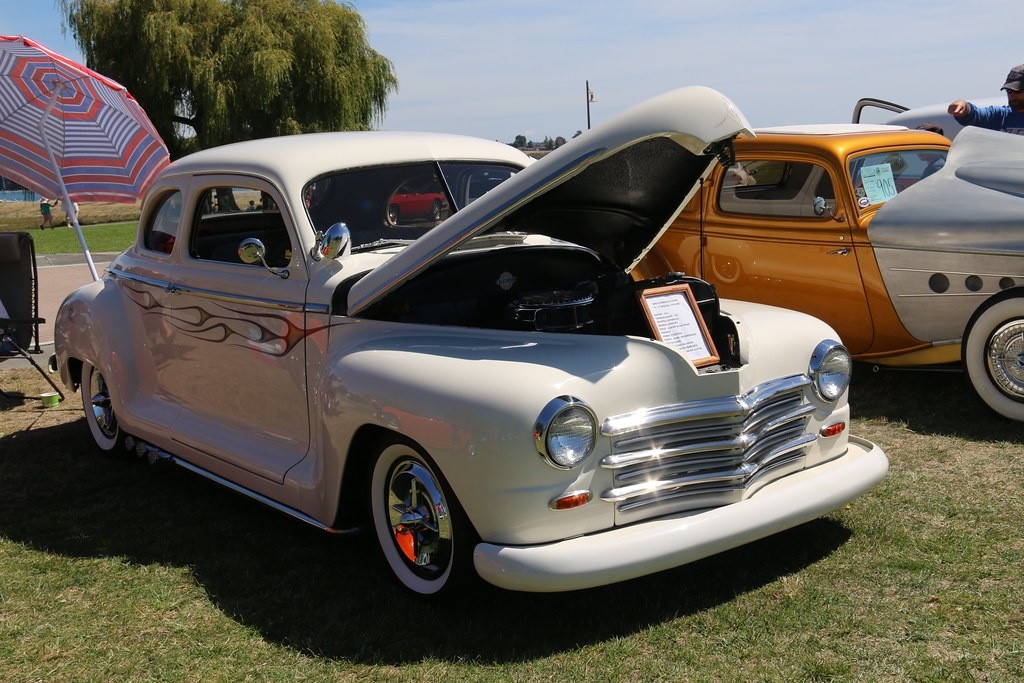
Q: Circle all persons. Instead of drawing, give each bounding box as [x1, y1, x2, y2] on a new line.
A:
[915, 122, 946, 179]
[948, 64, 1024, 136]
[67, 202, 79, 228]
[39, 197, 58, 230]
[0, 300, 17, 352]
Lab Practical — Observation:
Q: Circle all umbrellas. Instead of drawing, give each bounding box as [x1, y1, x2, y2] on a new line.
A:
[1, 34, 170, 283]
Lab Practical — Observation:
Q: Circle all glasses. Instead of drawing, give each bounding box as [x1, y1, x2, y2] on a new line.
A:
[1006, 88, 1023, 94]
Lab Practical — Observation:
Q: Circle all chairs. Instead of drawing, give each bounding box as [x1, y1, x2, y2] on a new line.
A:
[0, 231, 66, 403]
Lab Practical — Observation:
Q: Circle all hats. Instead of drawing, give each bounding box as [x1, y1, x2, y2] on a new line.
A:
[1000, 65, 1024, 91]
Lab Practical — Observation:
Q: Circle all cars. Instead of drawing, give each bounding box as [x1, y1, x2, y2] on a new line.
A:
[44, 81, 892, 607]
[625, 96, 1024, 426]
[383, 166, 517, 226]
